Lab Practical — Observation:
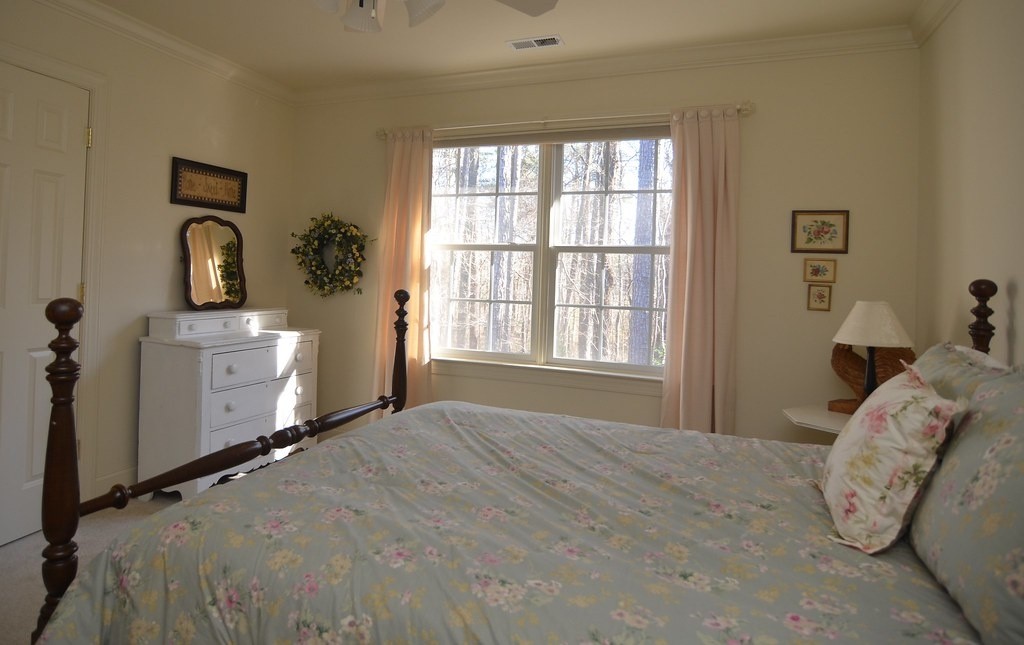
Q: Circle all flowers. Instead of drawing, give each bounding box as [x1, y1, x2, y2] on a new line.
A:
[289, 212, 379, 299]
[218, 237, 242, 302]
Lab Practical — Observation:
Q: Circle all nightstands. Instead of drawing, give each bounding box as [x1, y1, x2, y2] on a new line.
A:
[783, 403, 854, 433]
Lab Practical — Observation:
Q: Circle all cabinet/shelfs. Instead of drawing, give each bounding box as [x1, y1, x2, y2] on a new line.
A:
[137, 308, 323, 502]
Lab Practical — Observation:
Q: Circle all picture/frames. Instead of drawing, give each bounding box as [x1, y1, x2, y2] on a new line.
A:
[171, 157, 248, 213]
[804, 258, 837, 283]
[791, 210, 849, 254]
[808, 284, 832, 311]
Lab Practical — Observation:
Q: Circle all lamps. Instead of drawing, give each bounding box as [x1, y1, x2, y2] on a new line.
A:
[832, 300, 914, 397]
[313, 0, 445, 33]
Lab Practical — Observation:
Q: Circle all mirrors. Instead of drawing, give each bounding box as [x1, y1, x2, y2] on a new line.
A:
[181, 215, 247, 311]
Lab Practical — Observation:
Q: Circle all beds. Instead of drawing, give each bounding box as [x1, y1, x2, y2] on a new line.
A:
[27, 278, 1023, 645]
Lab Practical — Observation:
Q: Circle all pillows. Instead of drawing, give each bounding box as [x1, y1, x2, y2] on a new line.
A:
[806, 359, 970, 556]
[913, 339, 1010, 460]
[907, 372, 1023, 645]
